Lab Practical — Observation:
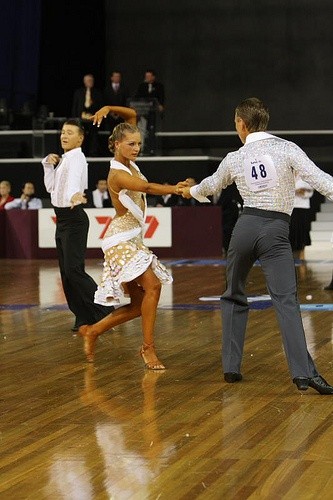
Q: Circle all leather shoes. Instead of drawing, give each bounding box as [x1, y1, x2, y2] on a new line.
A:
[224, 372, 243, 382]
[294, 375, 333, 393]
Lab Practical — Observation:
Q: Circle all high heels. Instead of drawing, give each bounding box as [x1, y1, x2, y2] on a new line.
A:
[71, 324, 96, 363]
[139, 342, 165, 371]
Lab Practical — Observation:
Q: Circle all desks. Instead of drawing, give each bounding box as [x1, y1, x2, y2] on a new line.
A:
[0, 206, 223, 259]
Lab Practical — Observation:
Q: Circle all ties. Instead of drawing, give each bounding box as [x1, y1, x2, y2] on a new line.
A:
[148, 83, 152, 93]
[85, 88, 91, 108]
[115, 83, 117, 90]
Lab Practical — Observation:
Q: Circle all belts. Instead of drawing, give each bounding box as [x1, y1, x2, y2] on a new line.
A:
[241, 206, 291, 223]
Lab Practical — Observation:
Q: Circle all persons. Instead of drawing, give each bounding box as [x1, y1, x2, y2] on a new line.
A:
[77, 106, 194, 371]
[92, 180, 110, 208]
[105, 72, 130, 134]
[42, 118, 116, 332]
[75, 75, 99, 156]
[157, 178, 225, 205]
[4, 182, 42, 209]
[0, 181, 14, 211]
[136, 71, 164, 155]
[178, 97, 333, 396]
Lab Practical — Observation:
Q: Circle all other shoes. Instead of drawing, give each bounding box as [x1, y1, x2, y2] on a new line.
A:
[149, 150, 156, 155]
[71, 328, 78, 331]
